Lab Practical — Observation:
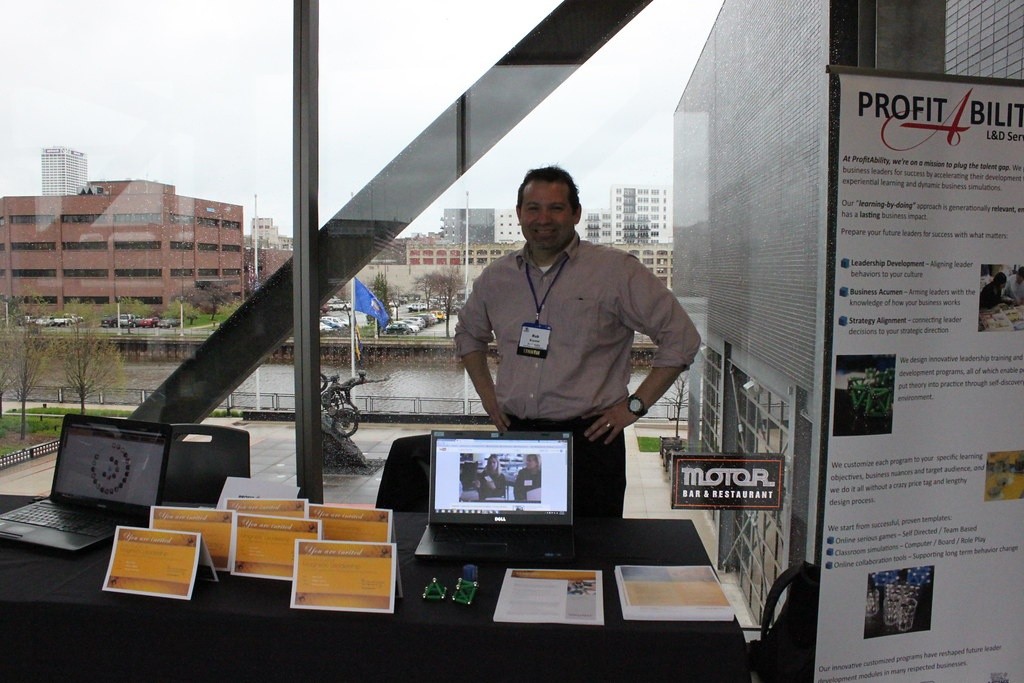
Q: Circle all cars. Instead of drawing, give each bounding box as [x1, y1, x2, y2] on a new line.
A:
[23, 312, 84, 328]
[382, 296, 466, 335]
[320, 295, 350, 313]
[101, 313, 180, 328]
[319, 316, 348, 331]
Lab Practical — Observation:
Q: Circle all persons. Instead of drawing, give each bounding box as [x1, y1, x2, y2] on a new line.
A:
[1001, 265, 1024, 305]
[453, 166, 702, 517]
[979, 271, 1010, 312]
[513, 454, 541, 501]
[477, 454, 505, 500]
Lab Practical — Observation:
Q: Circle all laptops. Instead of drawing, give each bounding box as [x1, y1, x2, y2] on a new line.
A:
[0, 414, 172, 555]
[414, 430, 576, 563]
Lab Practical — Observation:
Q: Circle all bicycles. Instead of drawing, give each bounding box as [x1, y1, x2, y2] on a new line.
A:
[320, 370, 361, 439]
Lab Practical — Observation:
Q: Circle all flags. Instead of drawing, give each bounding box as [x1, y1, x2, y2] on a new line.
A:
[354, 317, 363, 368]
[353, 278, 390, 328]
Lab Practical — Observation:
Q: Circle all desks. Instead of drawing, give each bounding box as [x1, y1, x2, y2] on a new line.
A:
[0, 495, 751, 683]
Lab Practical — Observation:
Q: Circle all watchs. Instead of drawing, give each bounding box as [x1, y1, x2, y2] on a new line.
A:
[626, 394, 647, 416]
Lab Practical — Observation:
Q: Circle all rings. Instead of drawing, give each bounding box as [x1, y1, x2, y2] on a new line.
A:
[605, 422, 611, 429]
